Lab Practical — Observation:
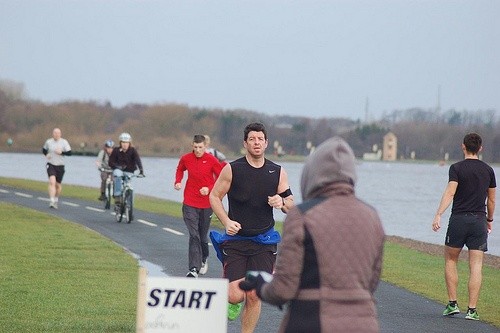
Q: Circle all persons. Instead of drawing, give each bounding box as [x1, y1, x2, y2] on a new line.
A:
[209, 123, 296, 333]
[96, 141, 116, 201]
[204, 135, 226, 162]
[254, 135, 384, 333]
[110, 133, 143, 221]
[432, 134, 496, 321]
[43, 127, 72, 209]
[174, 136, 222, 277]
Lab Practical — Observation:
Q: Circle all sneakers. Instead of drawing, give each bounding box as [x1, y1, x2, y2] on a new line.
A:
[464, 310, 479, 320]
[199, 259, 209, 276]
[55, 198, 59, 210]
[185, 267, 199, 279]
[442, 304, 460, 317]
[49, 200, 54, 208]
[227, 300, 244, 322]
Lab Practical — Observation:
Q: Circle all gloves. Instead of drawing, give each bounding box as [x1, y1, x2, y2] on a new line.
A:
[137, 171, 147, 180]
[117, 165, 128, 172]
[238, 274, 267, 304]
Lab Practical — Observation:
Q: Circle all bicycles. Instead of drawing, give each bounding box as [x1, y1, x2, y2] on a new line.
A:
[112, 171, 143, 223]
[98, 166, 119, 209]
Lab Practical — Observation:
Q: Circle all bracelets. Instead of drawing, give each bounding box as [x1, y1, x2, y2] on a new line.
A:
[281, 197, 285, 210]
[487, 218, 493, 222]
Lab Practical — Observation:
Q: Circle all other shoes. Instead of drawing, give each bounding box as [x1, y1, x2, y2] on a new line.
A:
[98, 194, 108, 202]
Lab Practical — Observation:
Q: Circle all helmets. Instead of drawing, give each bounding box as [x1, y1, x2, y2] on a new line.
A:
[119, 132, 133, 144]
[103, 140, 115, 149]
